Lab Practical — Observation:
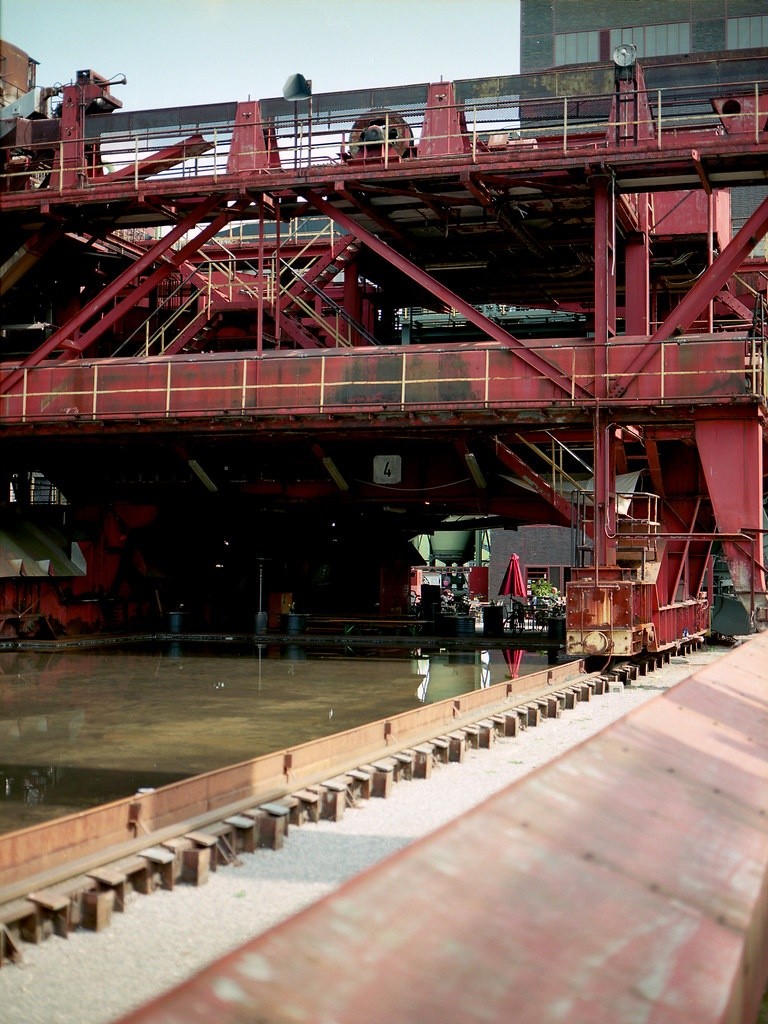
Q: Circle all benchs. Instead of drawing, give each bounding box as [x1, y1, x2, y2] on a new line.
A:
[305, 620, 435, 637]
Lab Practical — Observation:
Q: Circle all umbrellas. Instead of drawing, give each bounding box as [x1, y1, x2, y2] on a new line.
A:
[498, 552, 526, 621]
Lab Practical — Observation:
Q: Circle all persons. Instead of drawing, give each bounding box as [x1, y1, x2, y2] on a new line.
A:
[532, 586, 564, 621]
[440, 589, 454, 614]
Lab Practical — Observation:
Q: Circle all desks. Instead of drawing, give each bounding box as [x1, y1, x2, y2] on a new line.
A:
[306, 616, 363, 628]
[353, 614, 417, 633]
